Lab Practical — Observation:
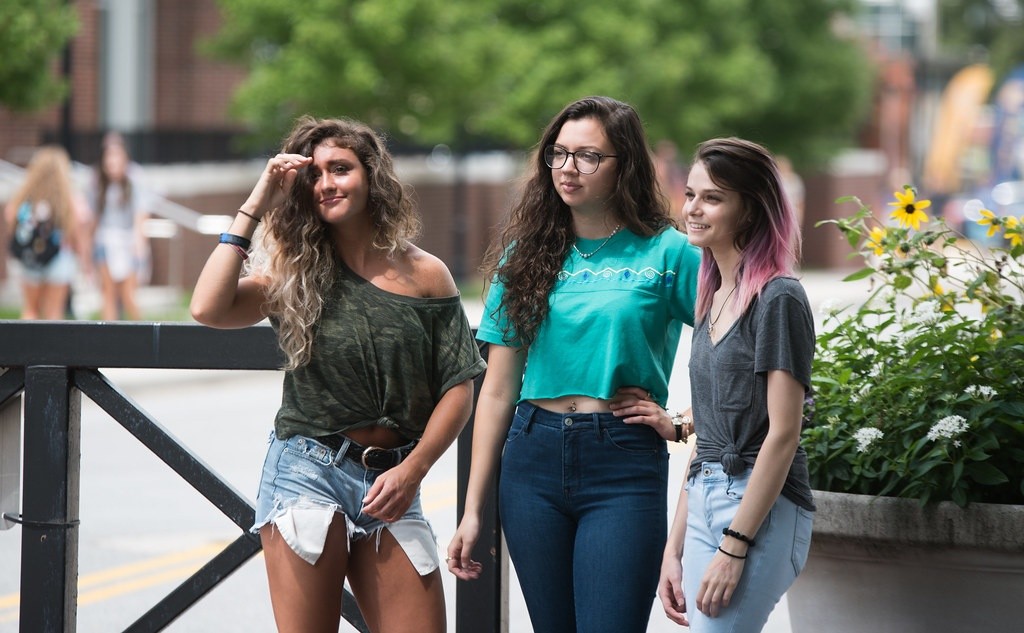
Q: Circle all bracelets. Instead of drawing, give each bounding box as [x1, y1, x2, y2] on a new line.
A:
[722, 527, 755, 547]
[677, 411, 691, 445]
[236, 208, 261, 223]
[219, 234, 251, 260]
[717, 546, 747, 559]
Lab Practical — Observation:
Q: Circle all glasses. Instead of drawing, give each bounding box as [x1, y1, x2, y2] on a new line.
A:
[544, 146, 620, 175]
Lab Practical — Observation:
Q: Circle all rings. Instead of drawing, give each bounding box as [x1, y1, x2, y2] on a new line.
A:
[446, 556, 457, 563]
[645, 392, 656, 401]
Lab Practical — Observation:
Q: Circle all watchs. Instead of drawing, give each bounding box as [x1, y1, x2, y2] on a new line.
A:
[671, 415, 683, 443]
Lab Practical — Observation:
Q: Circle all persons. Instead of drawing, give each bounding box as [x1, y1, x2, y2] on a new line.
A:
[0, 128, 150, 324]
[445, 93, 703, 633]
[658, 137, 817, 632]
[187, 113, 488, 633]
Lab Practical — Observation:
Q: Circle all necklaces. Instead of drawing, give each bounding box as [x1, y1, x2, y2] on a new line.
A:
[572, 224, 621, 259]
[708, 285, 737, 336]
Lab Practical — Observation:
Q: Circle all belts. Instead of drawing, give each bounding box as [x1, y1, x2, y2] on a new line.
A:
[318, 435, 414, 470]
